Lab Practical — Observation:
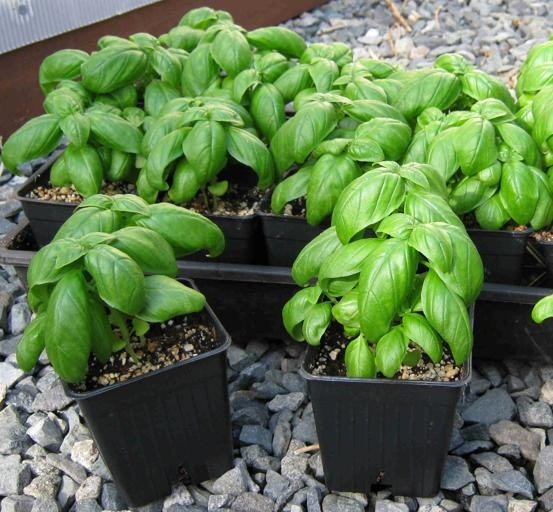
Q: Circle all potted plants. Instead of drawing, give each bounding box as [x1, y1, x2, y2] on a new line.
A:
[21, 194, 235, 503]
[286, 158, 475, 497]
[0, 8, 550, 289]
